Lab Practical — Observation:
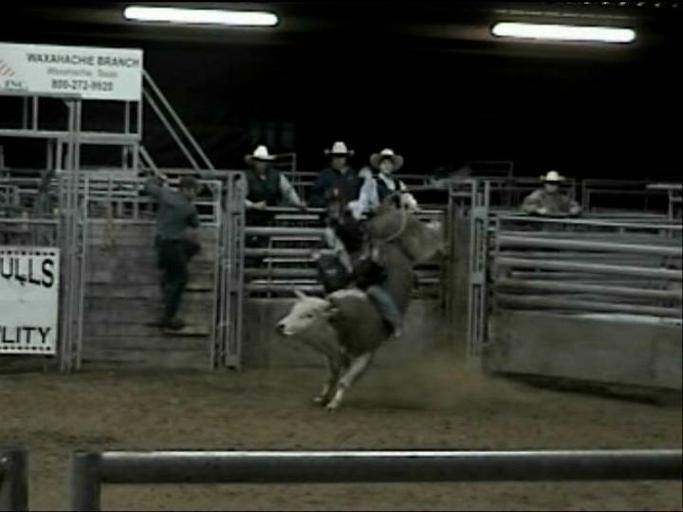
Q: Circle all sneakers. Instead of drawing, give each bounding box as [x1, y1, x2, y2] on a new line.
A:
[393, 313, 404, 337]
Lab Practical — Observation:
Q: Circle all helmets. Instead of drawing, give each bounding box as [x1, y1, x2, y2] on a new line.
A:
[321, 186, 347, 220]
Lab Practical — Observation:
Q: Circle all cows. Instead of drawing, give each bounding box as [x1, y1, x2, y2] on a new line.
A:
[274, 165, 473, 415]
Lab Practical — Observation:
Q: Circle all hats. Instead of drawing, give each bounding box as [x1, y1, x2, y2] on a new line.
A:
[540, 171, 566, 181]
[244, 146, 278, 164]
[370, 149, 405, 171]
[324, 141, 356, 154]
[180, 177, 205, 189]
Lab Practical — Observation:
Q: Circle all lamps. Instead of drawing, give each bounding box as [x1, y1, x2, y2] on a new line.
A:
[490, 9, 635, 44]
[122, 2, 280, 27]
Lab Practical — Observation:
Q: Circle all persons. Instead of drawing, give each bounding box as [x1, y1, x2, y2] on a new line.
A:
[353, 148, 419, 285]
[308, 187, 403, 344]
[237, 144, 308, 298]
[306, 140, 360, 206]
[142, 172, 202, 333]
[514, 170, 585, 272]
